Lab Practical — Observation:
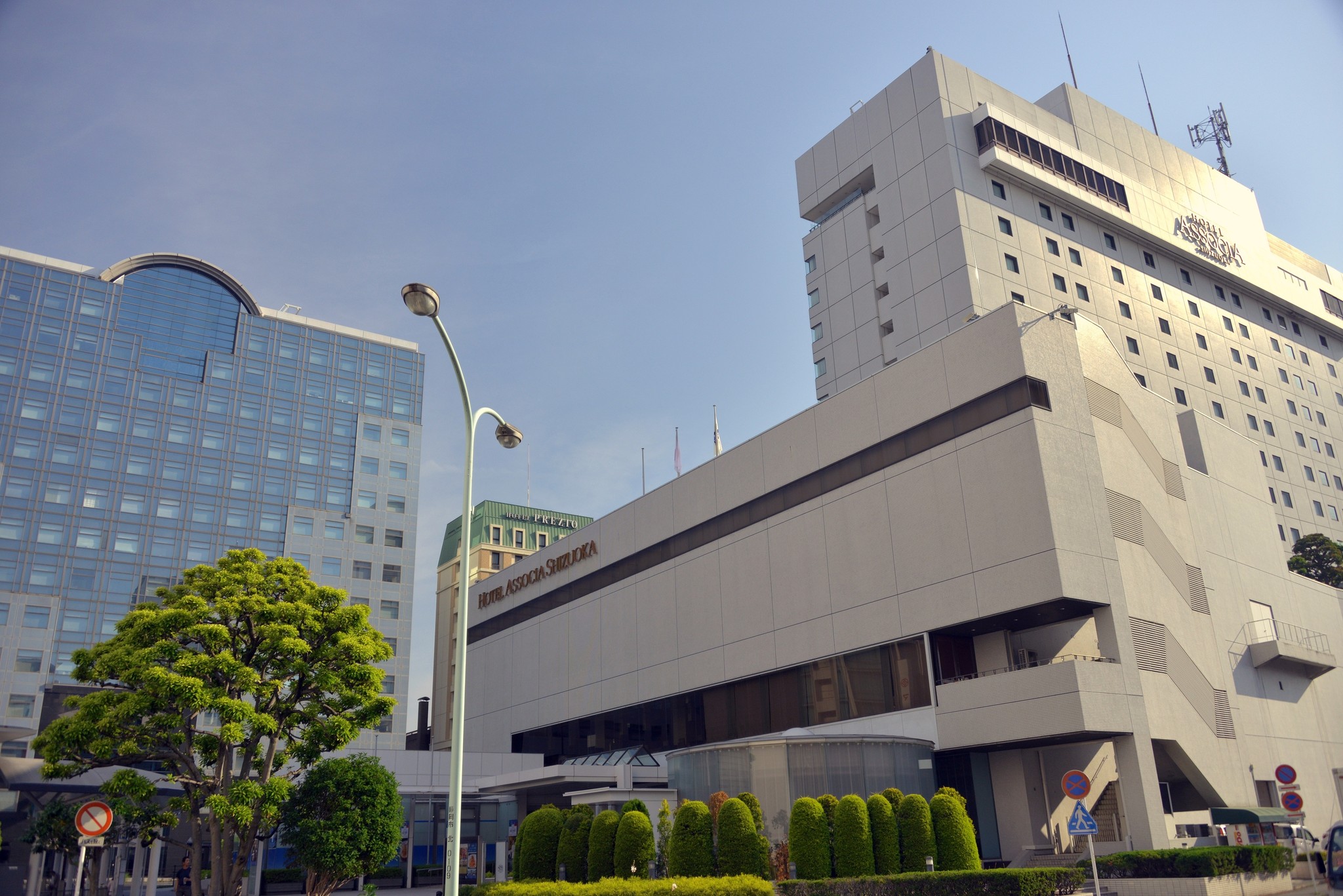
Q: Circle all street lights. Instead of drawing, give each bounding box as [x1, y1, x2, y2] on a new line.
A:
[402, 281, 526, 896]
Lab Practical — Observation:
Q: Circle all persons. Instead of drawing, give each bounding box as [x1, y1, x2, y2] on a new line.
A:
[46, 869, 60, 891]
[107, 877, 114, 896]
[174, 857, 192, 896]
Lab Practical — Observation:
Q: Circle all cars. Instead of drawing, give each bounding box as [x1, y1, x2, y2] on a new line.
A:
[1273, 822, 1324, 856]
[1313, 820, 1343, 896]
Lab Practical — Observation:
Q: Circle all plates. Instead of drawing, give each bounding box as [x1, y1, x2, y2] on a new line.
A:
[400, 841, 407, 858]
[468, 854, 476, 869]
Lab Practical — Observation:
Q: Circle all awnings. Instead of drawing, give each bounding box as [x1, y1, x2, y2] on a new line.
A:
[1208, 806, 1305, 846]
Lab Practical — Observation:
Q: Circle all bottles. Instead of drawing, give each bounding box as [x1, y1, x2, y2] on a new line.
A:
[183, 877, 186, 884]
